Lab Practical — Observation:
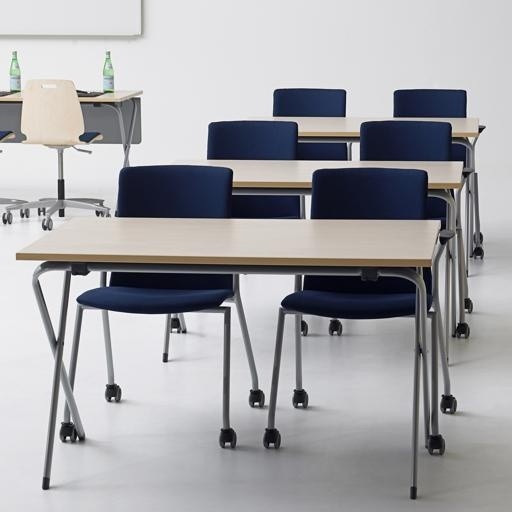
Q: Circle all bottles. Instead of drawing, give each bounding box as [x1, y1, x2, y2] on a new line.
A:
[101, 50, 114, 92]
[9, 51, 22, 92]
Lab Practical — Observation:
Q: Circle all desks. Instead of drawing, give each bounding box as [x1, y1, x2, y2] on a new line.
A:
[15, 218, 441, 501]
[0, 87, 143, 215]
[244, 113, 480, 172]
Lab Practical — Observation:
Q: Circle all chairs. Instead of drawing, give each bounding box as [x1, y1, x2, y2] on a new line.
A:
[2, 78, 110, 230]
